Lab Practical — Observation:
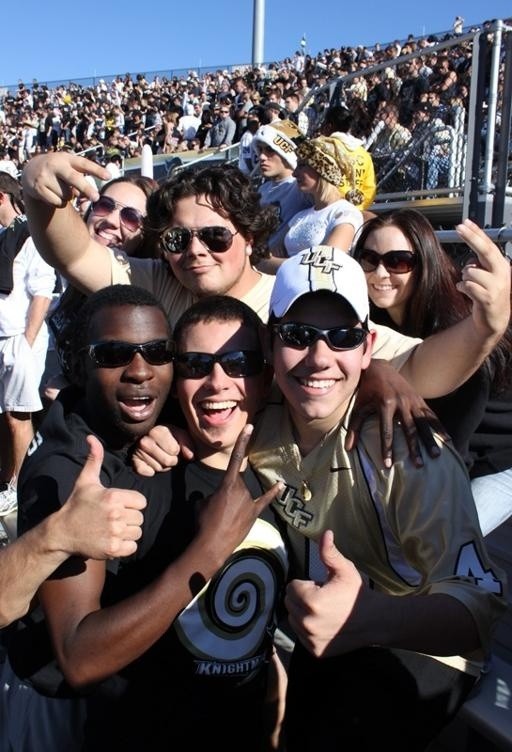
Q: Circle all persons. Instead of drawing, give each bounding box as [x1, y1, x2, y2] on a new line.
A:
[0, 25, 512, 752]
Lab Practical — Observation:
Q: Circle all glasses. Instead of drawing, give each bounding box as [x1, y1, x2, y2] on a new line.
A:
[271, 322, 367, 351]
[92, 195, 145, 227]
[79, 338, 173, 366]
[356, 249, 414, 274]
[160, 225, 238, 252]
[174, 349, 267, 379]
[219, 110, 228, 113]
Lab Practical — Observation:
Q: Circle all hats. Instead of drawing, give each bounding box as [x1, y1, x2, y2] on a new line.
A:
[295, 136, 364, 206]
[269, 245, 370, 332]
[252, 120, 300, 168]
